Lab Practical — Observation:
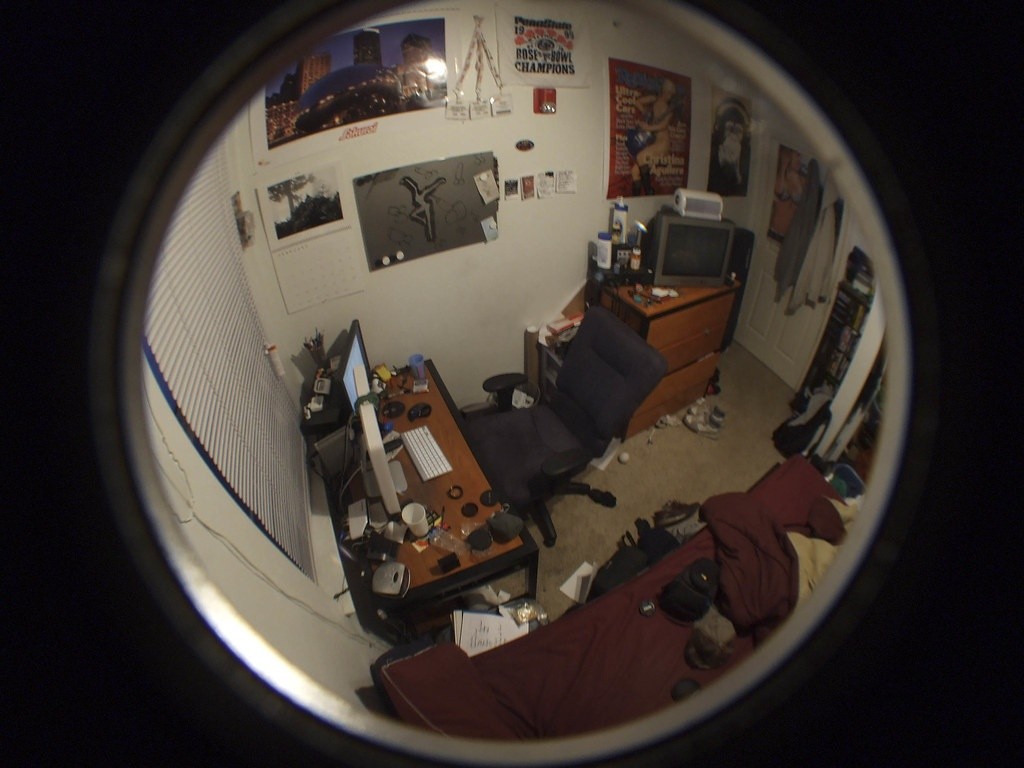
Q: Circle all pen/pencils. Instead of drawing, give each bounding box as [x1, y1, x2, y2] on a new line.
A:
[302, 327, 326, 350]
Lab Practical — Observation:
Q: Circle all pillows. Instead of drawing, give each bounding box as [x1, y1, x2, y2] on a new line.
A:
[379, 640, 519, 740]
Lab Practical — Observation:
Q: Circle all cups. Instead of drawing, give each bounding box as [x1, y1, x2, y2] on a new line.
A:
[410, 355, 426, 379]
[310, 346, 325, 364]
[402, 502, 428, 538]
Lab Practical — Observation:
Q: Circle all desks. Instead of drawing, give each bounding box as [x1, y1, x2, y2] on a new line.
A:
[316, 358, 539, 645]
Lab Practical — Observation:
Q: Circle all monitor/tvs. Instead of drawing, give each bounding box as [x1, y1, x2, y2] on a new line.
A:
[353, 369, 401, 524]
[337, 318, 376, 415]
[652, 210, 734, 288]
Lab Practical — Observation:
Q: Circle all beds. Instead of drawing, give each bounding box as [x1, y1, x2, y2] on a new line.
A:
[377, 451, 866, 741]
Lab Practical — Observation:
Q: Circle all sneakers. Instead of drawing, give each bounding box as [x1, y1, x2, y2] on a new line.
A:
[688, 405, 726, 428]
[653, 501, 700, 527]
[684, 413, 718, 441]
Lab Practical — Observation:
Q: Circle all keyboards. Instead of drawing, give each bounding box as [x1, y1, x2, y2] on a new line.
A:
[399, 427, 452, 483]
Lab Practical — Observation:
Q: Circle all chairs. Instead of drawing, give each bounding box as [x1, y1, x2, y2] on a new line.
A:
[457, 303, 671, 548]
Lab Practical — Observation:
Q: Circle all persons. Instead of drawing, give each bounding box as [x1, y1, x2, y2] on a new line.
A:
[765, 143, 807, 238]
[717, 119, 744, 195]
[630, 77, 676, 196]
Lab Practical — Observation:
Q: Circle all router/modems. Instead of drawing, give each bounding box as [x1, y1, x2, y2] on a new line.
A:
[315, 425, 358, 480]
[347, 499, 370, 540]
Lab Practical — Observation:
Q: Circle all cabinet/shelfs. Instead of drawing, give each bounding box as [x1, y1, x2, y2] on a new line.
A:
[598, 280, 742, 444]
[790, 256, 876, 417]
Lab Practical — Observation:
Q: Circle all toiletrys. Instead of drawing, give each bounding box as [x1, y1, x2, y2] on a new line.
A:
[635, 220, 647, 247]
[597, 232, 612, 269]
[613, 196, 628, 244]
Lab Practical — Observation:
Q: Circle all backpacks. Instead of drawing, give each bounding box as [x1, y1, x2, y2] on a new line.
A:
[772, 403, 832, 460]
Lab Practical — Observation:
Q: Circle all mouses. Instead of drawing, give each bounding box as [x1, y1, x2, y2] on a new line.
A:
[410, 401, 431, 423]
[385, 401, 405, 420]
